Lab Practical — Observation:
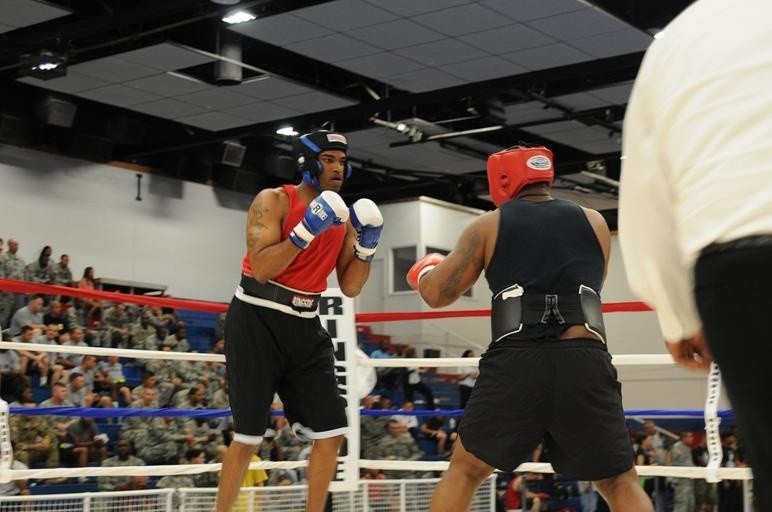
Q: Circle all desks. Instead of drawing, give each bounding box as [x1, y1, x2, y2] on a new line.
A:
[94, 276, 167, 297]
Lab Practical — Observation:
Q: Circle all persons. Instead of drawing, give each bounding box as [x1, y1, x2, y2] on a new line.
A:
[0, 291, 230, 510]
[496, 444, 602, 512]
[406, 144, 665, 512]
[619, 0, 772, 512]
[233, 392, 311, 512]
[213, 128, 384, 512]
[627, 420, 753, 512]
[357, 325, 479, 511]
[0, 233, 99, 291]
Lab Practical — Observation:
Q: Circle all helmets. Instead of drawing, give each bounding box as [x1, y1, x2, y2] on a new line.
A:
[486, 145, 555, 208]
[294, 129, 353, 191]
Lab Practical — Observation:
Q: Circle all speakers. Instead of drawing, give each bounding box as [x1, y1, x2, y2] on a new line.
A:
[46, 99, 78, 129]
[219, 142, 247, 168]
[275, 156, 298, 179]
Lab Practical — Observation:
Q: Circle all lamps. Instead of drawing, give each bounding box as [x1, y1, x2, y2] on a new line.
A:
[221, 7, 258, 25]
[31, 49, 61, 72]
[275, 125, 300, 137]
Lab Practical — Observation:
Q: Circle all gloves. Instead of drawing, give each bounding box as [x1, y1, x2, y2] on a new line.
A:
[406, 253, 446, 293]
[289, 190, 350, 250]
[350, 198, 384, 262]
